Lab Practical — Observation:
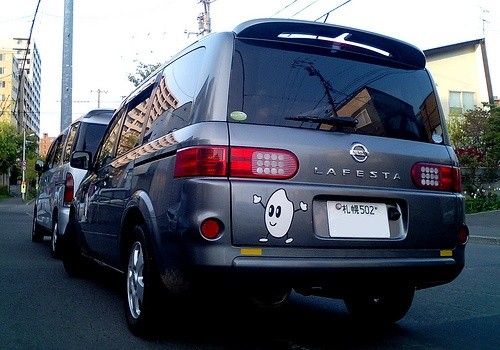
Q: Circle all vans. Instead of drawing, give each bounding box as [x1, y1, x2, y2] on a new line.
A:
[32, 108, 115, 260]
[63, 17, 469, 341]
